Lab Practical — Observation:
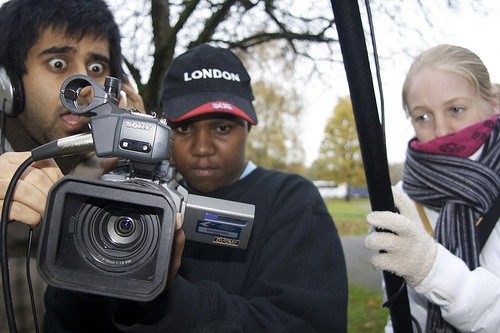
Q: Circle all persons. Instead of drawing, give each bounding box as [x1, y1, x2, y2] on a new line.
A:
[363, 44, 500, 333]
[42, 45, 348, 333]
[0, 0, 147, 333]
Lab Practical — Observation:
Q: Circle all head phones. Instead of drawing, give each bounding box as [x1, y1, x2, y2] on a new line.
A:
[0, 63, 26, 117]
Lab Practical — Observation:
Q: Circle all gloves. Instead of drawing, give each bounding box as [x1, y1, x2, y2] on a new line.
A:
[364, 185, 438, 288]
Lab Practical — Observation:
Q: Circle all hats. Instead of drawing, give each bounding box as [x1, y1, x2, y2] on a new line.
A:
[158, 44, 258, 125]
